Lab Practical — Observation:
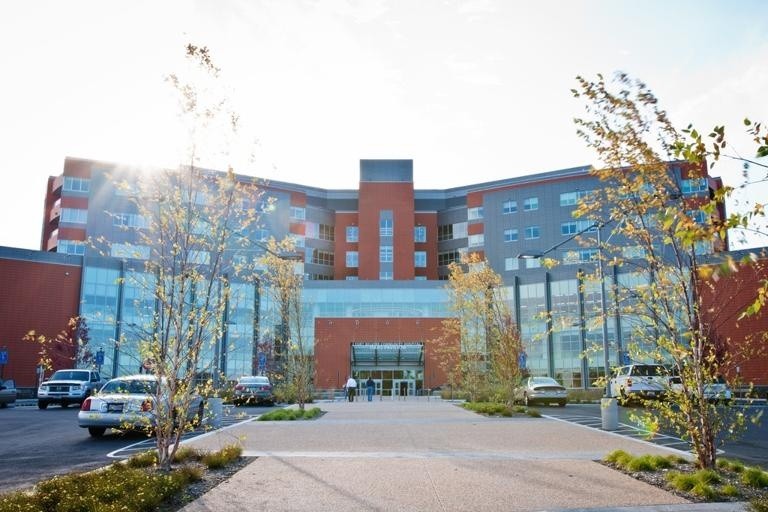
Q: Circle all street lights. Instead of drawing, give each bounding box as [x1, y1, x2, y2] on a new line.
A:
[514, 189, 682, 399]
[152, 195, 301, 460]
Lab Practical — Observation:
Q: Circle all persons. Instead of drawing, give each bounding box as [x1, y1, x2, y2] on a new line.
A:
[346, 377, 357, 402]
[365, 376, 375, 402]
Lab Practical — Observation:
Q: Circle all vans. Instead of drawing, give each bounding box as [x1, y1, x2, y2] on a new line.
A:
[609, 363, 668, 403]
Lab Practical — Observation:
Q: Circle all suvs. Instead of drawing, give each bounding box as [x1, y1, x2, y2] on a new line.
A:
[38, 368, 108, 410]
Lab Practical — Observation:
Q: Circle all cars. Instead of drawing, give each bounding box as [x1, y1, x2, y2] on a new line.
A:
[511, 375, 566, 406]
[703, 377, 732, 404]
[233, 377, 274, 407]
[77, 374, 204, 439]
[1, 378, 16, 407]
[667, 375, 684, 393]
